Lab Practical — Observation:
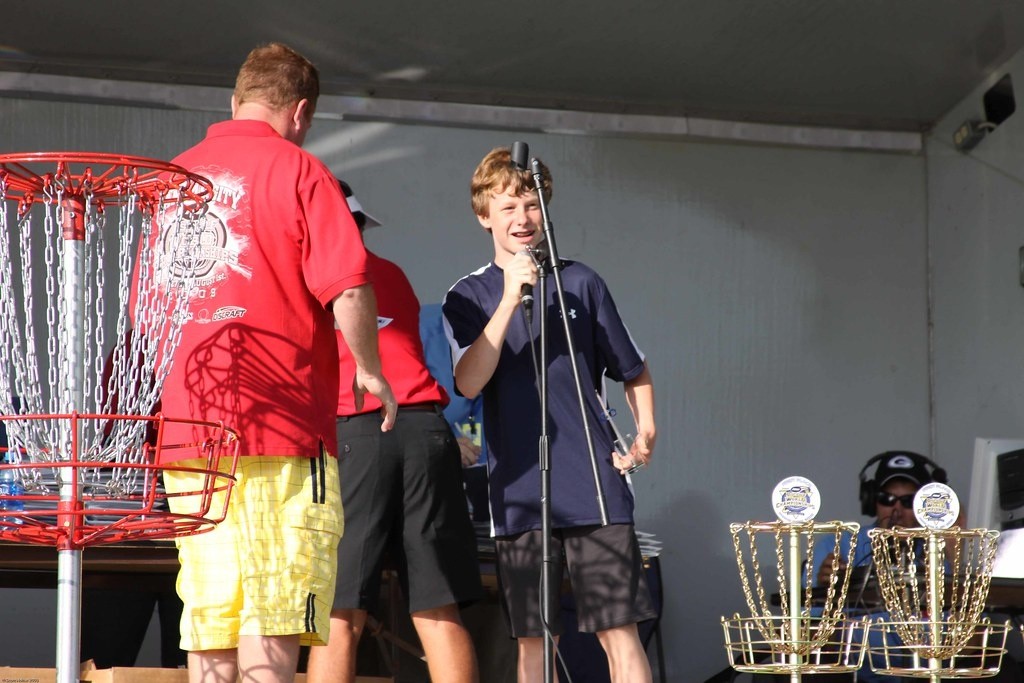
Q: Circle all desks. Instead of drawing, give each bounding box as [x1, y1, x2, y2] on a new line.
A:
[0, 518, 665, 683]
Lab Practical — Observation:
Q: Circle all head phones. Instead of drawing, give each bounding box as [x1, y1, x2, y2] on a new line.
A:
[859, 450, 948, 517]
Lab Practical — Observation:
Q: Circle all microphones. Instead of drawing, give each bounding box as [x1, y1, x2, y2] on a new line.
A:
[515, 250, 535, 324]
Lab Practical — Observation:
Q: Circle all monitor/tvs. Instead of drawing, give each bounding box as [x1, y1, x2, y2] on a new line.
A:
[964, 437, 1024, 579]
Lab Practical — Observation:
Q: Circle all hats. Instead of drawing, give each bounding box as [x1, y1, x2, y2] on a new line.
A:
[875, 451, 930, 491]
[345, 196, 381, 229]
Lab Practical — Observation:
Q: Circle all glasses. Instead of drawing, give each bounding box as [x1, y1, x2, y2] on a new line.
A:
[876, 491, 915, 508]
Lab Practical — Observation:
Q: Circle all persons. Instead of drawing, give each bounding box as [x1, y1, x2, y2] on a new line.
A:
[440, 147, 657, 683]
[308, 178, 491, 683]
[82, 42, 398, 683]
[797, 449, 967, 647]
